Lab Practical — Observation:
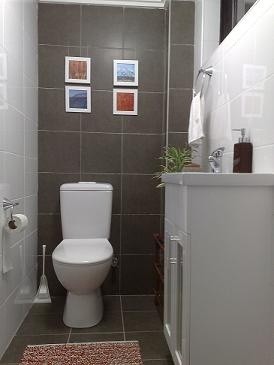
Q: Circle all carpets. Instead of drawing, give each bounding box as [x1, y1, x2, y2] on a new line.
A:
[21, 339, 141, 363]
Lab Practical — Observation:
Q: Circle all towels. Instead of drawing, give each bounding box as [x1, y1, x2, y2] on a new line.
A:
[188, 89, 205, 144]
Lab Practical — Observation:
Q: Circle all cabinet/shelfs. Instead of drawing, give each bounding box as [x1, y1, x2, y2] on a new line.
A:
[162, 173, 273, 365]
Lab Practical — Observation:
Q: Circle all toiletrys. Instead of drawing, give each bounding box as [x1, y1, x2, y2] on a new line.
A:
[231, 127, 254, 174]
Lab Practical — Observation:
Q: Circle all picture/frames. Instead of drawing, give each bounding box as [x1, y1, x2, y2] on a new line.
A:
[113, 89, 138, 116]
[65, 57, 90, 84]
[64, 86, 92, 113]
[113, 59, 138, 86]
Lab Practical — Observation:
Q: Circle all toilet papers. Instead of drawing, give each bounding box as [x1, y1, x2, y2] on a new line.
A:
[2, 214, 30, 272]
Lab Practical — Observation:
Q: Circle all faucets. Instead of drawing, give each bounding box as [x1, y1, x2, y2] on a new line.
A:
[207, 146, 226, 174]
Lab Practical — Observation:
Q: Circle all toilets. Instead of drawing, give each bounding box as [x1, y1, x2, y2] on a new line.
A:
[51, 181, 114, 329]
